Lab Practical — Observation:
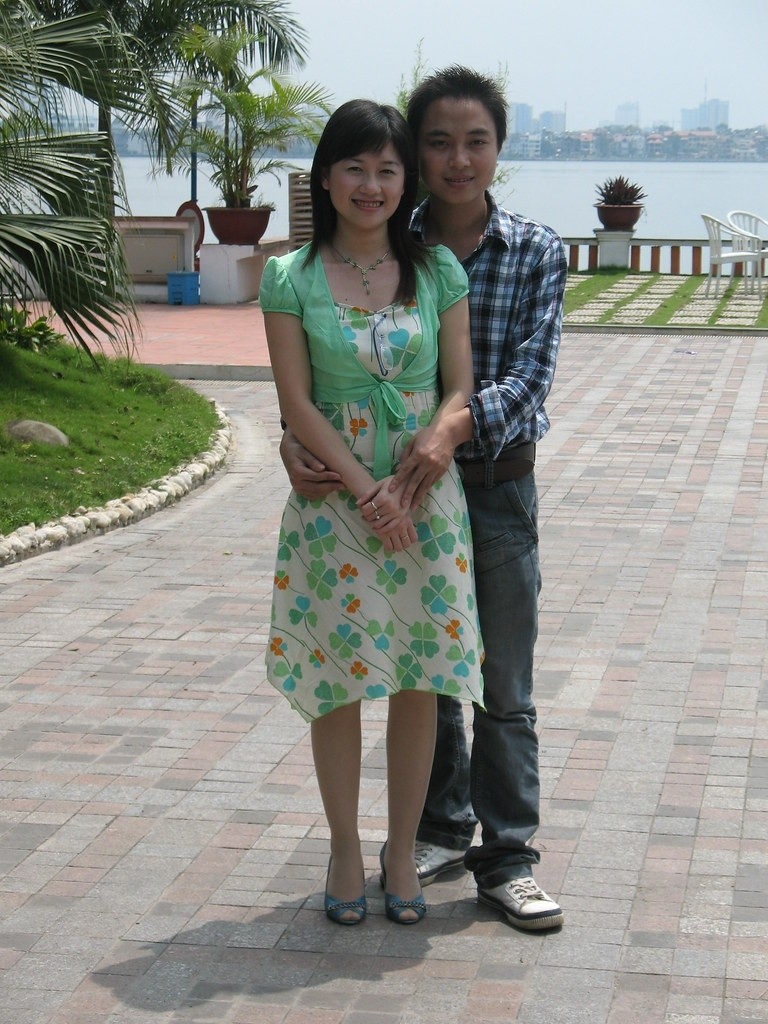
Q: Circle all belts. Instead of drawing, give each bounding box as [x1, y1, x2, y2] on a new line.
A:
[454, 442, 535, 486]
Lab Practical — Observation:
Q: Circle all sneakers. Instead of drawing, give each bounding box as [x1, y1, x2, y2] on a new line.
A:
[380, 840, 467, 888]
[477, 874, 564, 929]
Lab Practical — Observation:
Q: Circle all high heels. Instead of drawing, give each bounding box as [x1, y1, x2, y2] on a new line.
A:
[324, 851, 367, 925]
[380, 838, 428, 925]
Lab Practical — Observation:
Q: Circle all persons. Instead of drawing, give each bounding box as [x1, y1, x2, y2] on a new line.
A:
[260, 63, 568, 931]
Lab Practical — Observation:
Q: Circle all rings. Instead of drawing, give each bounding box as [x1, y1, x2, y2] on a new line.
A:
[375, 511, 380, 520]
[371, 501, 378, 510]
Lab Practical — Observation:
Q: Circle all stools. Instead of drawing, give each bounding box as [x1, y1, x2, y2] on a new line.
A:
[165, 270, 201, 307]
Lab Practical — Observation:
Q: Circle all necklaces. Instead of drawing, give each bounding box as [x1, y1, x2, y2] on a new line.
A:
[329, 241, 392, 295]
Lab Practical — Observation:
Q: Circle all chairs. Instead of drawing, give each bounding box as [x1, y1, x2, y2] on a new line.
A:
[701, 211, 768, 299]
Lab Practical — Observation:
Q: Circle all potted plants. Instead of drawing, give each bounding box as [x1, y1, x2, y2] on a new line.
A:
[146, 21, 338, 245]
[591, 175, 649, 232]
